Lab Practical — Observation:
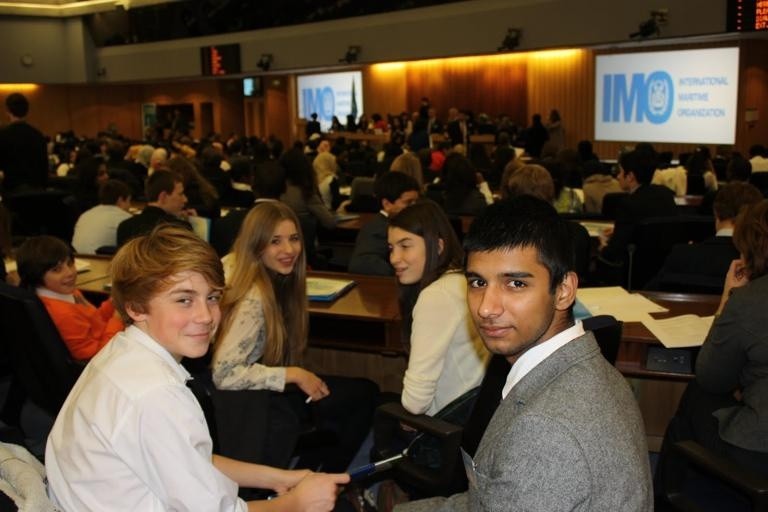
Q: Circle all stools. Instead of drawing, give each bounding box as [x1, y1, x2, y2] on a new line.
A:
[669, 437, 767, 510]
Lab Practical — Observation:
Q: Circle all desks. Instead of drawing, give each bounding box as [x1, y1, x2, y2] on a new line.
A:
[0, 127, 729, 383]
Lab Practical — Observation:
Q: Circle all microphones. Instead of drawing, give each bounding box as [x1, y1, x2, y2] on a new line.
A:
[628, 244, 635, 293]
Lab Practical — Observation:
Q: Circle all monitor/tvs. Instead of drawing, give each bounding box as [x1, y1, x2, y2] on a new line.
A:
[243, 78, 262, 97]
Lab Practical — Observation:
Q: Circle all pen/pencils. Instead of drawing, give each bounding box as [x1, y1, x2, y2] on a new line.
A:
[305, 397, 313, 404]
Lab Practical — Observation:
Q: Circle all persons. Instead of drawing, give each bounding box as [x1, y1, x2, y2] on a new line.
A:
[0, 81, 767, 512]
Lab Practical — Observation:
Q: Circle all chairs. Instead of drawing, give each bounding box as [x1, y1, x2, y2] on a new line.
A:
[1, 282, 90, 421]
[184, 355, 348, 478]
[368, 312, 624, 498]
